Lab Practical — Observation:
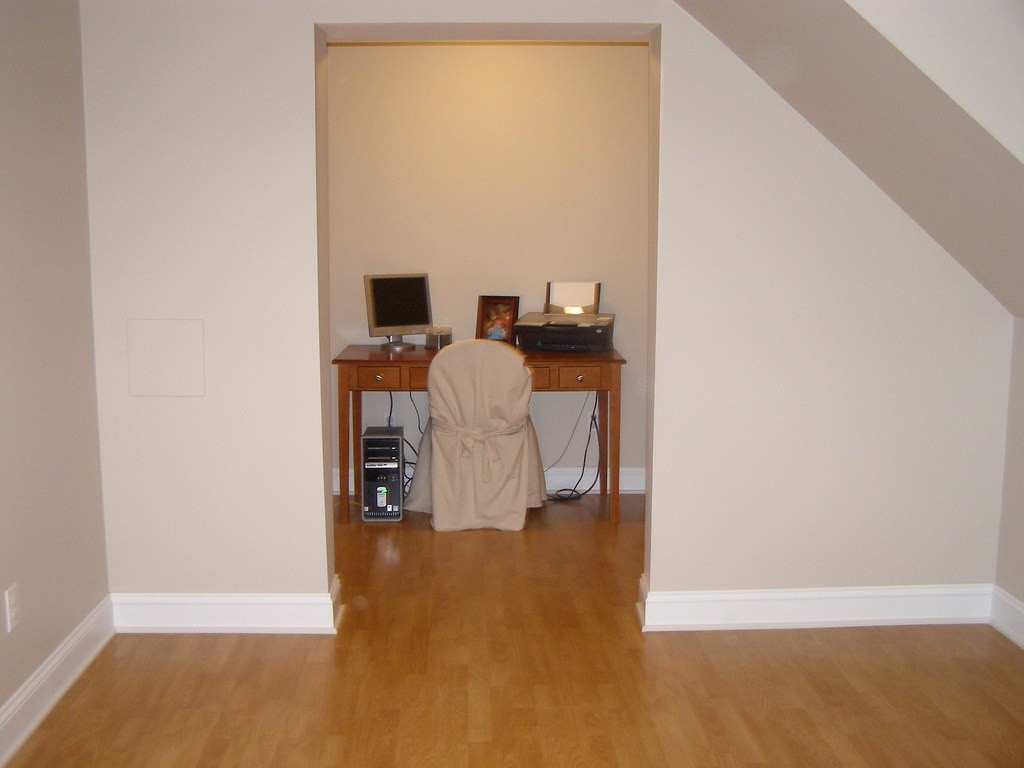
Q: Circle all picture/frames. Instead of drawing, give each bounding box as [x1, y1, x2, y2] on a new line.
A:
[475, 296, 519, 348]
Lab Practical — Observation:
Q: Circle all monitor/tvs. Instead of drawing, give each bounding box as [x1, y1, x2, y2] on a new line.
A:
[364, 273, 433, 351]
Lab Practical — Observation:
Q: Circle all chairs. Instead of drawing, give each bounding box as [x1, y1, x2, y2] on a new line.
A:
[403, 338, 547, 530]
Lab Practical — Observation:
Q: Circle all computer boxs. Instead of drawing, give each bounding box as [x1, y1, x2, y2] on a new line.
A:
[361, 426, 405, 522]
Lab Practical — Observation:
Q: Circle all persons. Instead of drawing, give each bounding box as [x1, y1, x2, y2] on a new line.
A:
[484, 310, 509, 340]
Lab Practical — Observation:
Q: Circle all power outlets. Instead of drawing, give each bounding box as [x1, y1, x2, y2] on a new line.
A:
[586, 409, 600, 429]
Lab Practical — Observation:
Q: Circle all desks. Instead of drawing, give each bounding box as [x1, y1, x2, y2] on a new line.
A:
[331, 343, 625, 526]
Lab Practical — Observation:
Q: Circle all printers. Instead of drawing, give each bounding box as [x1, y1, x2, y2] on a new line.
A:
[513, 281, 616, 353]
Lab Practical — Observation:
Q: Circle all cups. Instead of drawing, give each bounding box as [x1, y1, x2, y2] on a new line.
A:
[440, 326, 452, 349]
[426, 326, 438, 348]
